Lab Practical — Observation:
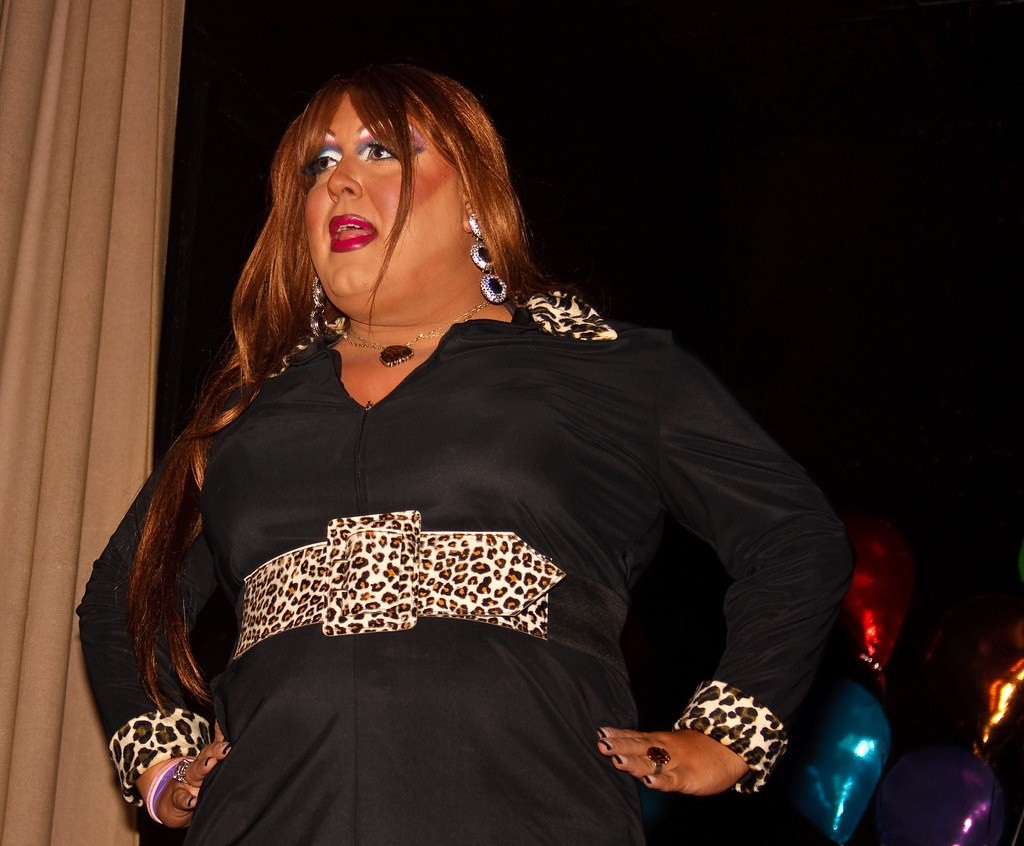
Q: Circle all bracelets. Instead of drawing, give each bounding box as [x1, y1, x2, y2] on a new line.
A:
[146, 756, 196, 825]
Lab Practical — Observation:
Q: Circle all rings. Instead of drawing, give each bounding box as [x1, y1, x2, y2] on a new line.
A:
[173, 759, 189, 783]
[647, 747, 669, 775]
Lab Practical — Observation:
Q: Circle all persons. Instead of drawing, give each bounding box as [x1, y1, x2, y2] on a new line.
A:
[78, 63, 857, 846]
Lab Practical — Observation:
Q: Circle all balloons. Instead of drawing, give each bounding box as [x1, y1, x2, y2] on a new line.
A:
[643, 518, 1023, 846]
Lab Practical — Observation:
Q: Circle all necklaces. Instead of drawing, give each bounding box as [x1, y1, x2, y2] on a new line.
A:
[338, 299, 489, 367]
